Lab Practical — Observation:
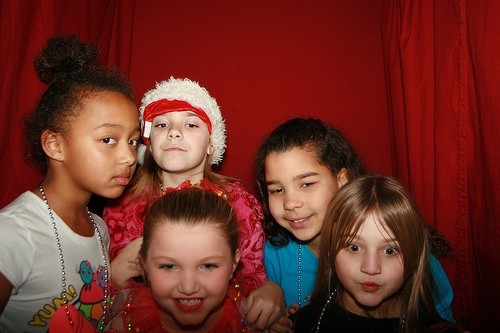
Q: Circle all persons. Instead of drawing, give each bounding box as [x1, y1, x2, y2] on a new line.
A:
[100, 74, 284, 329]
[0, 32, 141, 333]
[253, 118, 456, 333]
[49, 186, 270, 333]
[288, 176, 463, 333]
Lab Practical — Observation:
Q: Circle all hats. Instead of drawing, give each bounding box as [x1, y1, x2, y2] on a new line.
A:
[137, 75, 228, 165]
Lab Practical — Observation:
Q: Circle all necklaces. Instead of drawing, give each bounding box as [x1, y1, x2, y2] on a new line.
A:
[298, 240, 302, 307]
[317, 289, 405, 333]
[40, 180, 108, 333]
